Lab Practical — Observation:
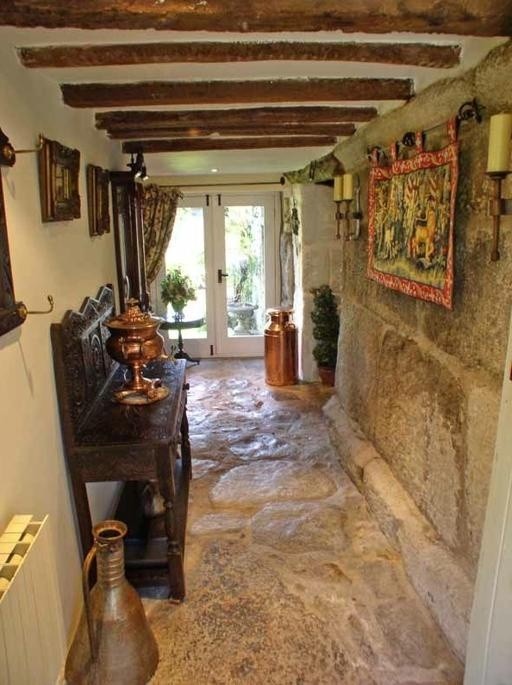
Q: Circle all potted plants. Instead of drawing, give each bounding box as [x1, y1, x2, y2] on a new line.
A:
[161, 269, 191, 364]
[228, 222, 258, 335]
[312, 284, 338, 386]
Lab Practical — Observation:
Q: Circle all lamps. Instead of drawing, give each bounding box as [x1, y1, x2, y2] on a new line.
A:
[127, 149, 149, 182]
[332, 173, 361, 241]
[486, 114, 511, 260]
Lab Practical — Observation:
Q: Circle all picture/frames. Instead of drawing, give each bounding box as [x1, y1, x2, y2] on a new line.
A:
[365, 141, 461, 308]
[87, 163, 111, 236]
[40, 136, 81, 222]
[0, 130, 28, 335]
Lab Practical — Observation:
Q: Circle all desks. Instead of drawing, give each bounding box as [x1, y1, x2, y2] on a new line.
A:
[50, 284, 192, 599]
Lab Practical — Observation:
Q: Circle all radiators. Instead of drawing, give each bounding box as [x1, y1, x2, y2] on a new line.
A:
[0, 513, 65, 684]
